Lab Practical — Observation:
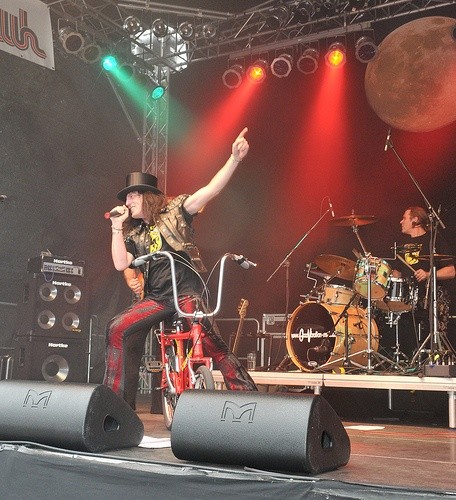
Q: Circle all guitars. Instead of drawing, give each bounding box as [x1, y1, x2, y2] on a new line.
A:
[233, 298, 248, 354]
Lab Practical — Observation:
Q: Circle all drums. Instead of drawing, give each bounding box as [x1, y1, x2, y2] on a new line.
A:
[320, 284, 361, 307]
[371, 278, 418, 311]
[352, 257, 393, 301]
[285, 301, 379, 373]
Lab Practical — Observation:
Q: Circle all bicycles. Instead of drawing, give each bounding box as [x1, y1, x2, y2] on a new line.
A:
[127, 249, 259, 429]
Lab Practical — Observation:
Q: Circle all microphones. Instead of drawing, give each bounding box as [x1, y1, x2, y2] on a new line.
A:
[328, 197, 334, 218]
[384, 129, 391, 151]
[129, 253, 158, 269]
[104, 211, 119, 219]
[231, 254, 250, 269]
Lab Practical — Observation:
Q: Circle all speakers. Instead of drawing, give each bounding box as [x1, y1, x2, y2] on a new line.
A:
[215, 318, 260, 359]
[0, 378, 144, 454]
[10, 337, 88, 382]
[16, 274, 88, 339]
[170, 389, 350, 475]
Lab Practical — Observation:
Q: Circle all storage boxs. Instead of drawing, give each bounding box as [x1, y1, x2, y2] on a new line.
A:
[260, 313, 302, 372]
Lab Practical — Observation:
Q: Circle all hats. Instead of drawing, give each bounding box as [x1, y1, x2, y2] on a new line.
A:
[117, 172, 164, 201]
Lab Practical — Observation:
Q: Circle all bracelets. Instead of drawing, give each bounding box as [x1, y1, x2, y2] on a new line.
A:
[111, 225, 124, 232]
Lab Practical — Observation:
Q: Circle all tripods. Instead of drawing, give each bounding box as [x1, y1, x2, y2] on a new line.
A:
[312, 146, 456, 376]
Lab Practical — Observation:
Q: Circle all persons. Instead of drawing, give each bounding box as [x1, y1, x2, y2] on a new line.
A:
[100, 127, 258, 410]
[369, 203, 456, 370]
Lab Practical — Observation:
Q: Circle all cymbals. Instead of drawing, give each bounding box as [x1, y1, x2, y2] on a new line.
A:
[327, 215, 379, 227]
[314, 254, 357, 281]
[415, 254, 456, 261]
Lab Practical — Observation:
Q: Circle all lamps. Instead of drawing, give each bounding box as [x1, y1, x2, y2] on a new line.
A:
[291, 0, 316, 23]
[117, 56, 136, 82]
[221, 57, 246, 89]
[270, 45, 295, 78]
[101, 50, 120, 73]
[265, 4, 295, 30]
[246, 52, 270, 85]
[152, 17, 169, 38]
[296, 39, 321, 75]
[202, 22, 217, 40]
[56, 16, 85, 57]
[144, 76, 166, 101]
[320, 0, 350, 17]
[324, 33, 347, 71]
[123, 9, 142, 36]
[77, 34, 104, 66]
[177, 21, 196, 41]
[353, 28, 379, 64]
